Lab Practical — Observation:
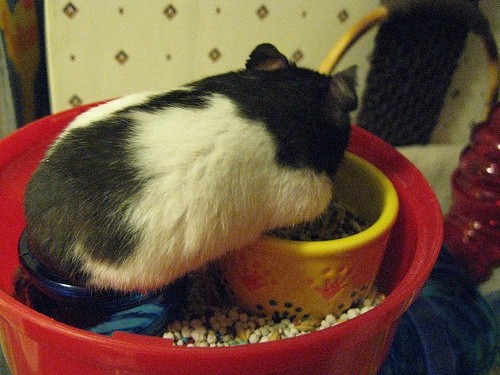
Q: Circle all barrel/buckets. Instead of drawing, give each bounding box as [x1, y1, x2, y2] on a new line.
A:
[1, 96, 444, 375]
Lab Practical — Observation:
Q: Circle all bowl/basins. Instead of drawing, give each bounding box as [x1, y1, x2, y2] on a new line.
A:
[225, 151, 398, 320]
